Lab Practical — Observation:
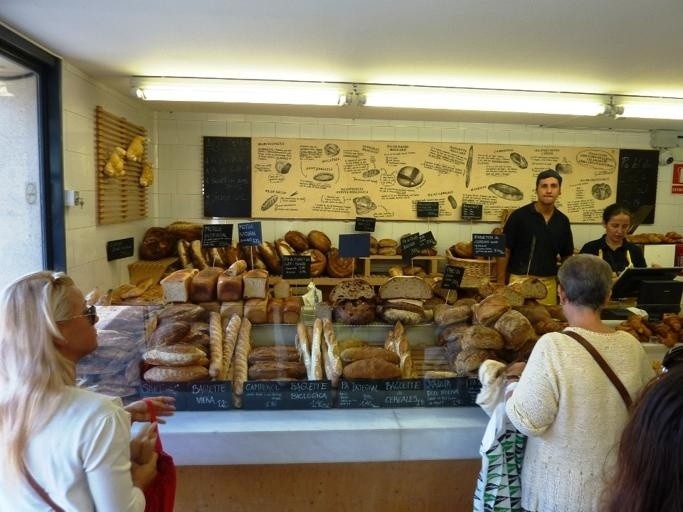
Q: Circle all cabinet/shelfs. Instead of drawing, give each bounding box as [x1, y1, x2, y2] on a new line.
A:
[269, 253, 447, 287]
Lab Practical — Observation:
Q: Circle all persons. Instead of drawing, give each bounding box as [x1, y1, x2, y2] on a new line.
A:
[502, 254, 656, 512]
[1, 268, 178, 512]
[596, 359, 683, 512]
[576, 201, 645, 271]
[492, 168, 575, 304]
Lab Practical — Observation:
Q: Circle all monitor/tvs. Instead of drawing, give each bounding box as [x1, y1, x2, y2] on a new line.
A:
[610, 267, 683, 298]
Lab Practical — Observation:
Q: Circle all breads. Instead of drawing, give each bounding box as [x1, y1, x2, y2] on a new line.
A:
[626, 231, 683, 243]
[75, 221, 566, 408]
[615, 313, 683, 347]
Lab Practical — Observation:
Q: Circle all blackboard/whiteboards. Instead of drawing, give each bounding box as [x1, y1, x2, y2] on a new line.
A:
[203, 135, 660, 225]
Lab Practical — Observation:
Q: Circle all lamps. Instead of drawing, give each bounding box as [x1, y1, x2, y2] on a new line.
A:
[337, 85, 367, 107]
[603, 97, 625, 119]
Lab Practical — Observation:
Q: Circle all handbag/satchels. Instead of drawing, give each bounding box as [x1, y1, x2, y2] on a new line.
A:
[472, 382, 528, 512]
[142, 398, 176, 512]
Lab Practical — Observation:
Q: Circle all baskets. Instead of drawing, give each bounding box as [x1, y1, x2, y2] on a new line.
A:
[445, 249, 498, 281]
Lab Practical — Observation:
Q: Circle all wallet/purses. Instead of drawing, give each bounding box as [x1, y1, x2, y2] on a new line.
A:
[130, 421, 158, 465]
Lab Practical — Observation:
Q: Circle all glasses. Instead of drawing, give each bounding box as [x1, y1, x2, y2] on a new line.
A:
[69, 305, 99, 325]
